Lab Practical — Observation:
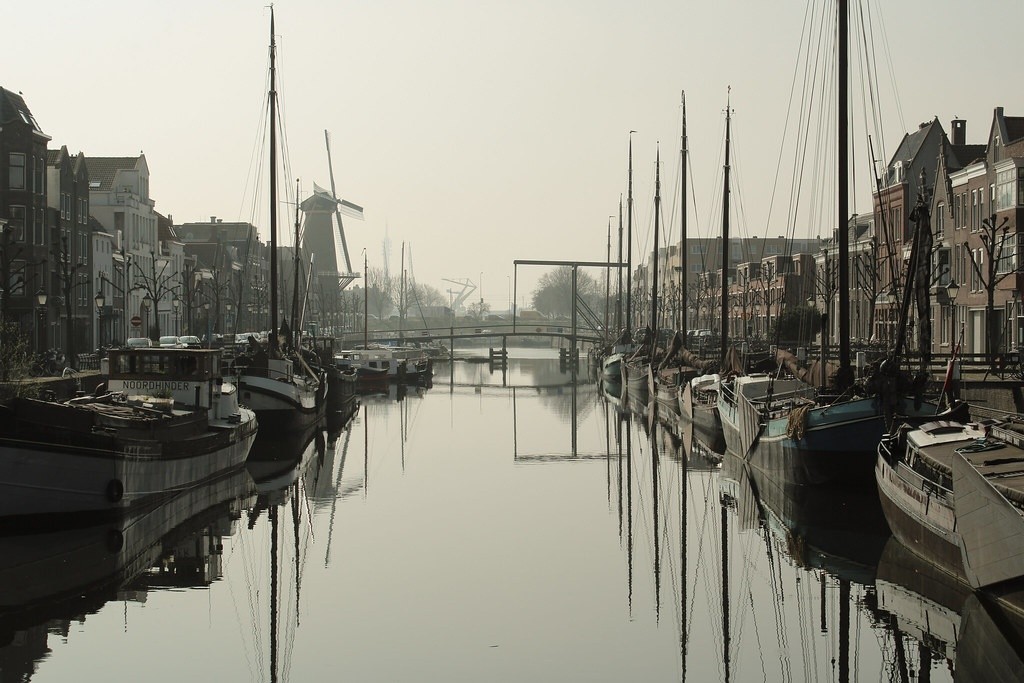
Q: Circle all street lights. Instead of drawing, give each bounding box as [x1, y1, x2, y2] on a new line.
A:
[701, 304, 707, 329]
[95, 291, 105, 348]
[688, 305, 694, 329]
[946, 279, 960, 352]
[225, 300, 232, 333]
[247, 303, 254, 331]
[203, 299, 210, 338]
[172, 296, 179, 335]
[807, 296, 816, 356]
[716, 303, 722, 333]
[731, 302, 740, 335]
[754, 300, 760, 335]
[263, 306, 268, 330]
[886, 286, 895, 359]
[143, 293, 152, 337]
[36, 286, 48, 353]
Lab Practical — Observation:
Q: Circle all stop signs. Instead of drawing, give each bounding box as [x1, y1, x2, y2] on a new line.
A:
[130, 316, 142, 326]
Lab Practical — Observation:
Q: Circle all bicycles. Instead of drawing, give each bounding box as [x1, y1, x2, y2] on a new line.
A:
[28, 348, 65, 377]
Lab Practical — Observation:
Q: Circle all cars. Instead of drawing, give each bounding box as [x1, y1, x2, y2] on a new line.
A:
[126, 338, 153, 348]
[159, 336, 201, 346]
[202, 328, 313, 344]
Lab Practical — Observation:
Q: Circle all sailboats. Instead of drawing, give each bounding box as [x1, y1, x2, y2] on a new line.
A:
[713, 451, 885, 683]
[225, 2, 435, 464]
[593, 0, 973, 494]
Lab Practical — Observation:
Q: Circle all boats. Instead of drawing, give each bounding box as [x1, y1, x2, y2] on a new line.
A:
[875, 399, 1024, 618]
[0, 464, 258, 640]
[0, 344, 259, 522]
[869, 537, 1024, 683]
